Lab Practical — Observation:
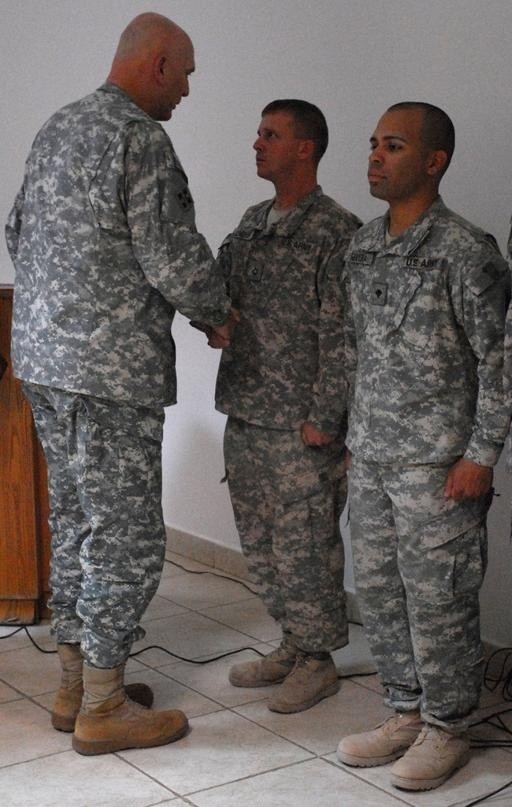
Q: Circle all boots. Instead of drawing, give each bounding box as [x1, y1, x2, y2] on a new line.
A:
[72, 663, 188, 755]
[52, 643, 153, 731]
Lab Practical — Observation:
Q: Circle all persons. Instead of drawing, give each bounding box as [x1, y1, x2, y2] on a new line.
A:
[2, 9, 239, 755]
[217, 100, 364, 711]
[340, 103, 511, 792]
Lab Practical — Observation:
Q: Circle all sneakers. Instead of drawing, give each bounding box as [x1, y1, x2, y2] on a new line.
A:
[338, 710, 424, 767]
[269, 652, 339, 714]
[391, 723, 472, 790]
[229, 643, 300, 688]
